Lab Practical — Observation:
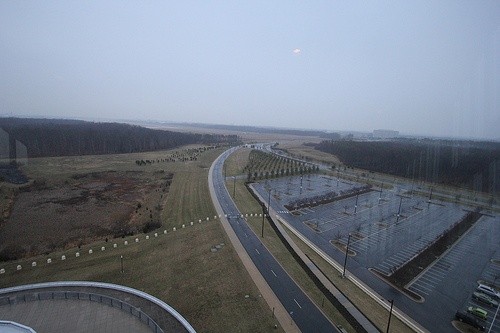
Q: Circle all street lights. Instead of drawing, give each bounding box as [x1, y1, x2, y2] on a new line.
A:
[299, 175, 303, 194]
[343, 232, 352, 276]
[386, 299, 394, 332]
[258, 201, 265, 238]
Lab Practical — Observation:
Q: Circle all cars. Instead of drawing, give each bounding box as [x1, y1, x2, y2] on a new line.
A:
[456, 310, 487, 330]
[472, 291, 499, 307]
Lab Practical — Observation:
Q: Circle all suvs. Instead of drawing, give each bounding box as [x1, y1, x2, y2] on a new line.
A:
[468, 307, 488, 320]
[477, 285, 500, 300]
